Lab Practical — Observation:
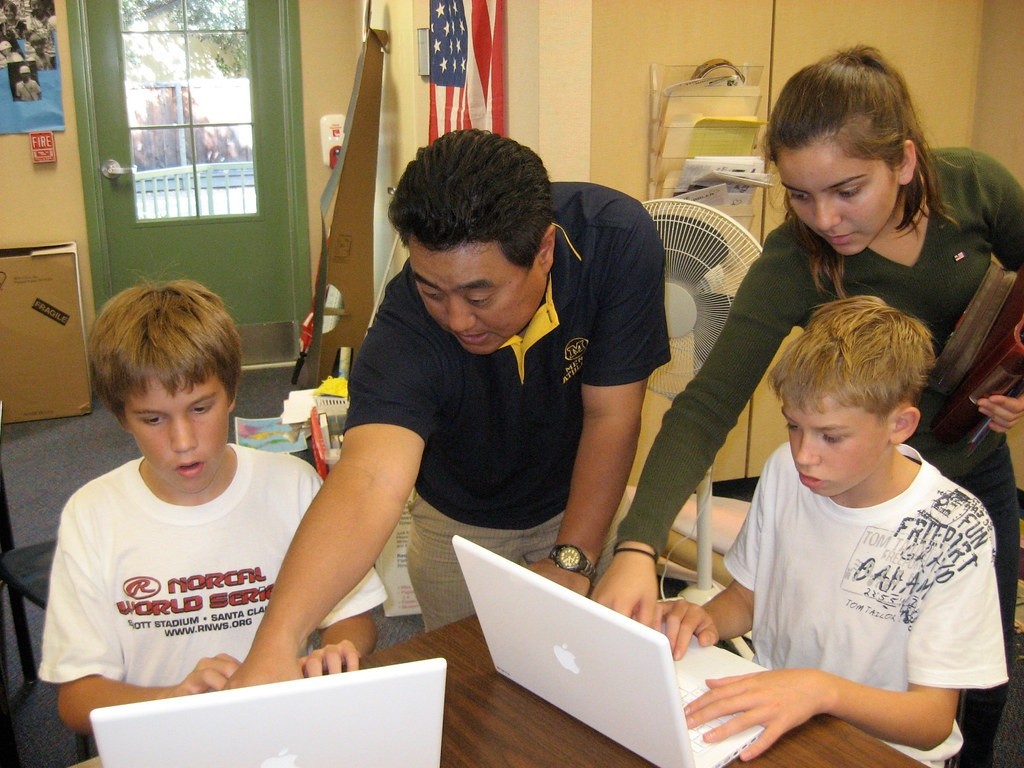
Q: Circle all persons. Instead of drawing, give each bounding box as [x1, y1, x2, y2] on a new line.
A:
[0, 0, 56, 102]
[221, 129, 671, 693]
[592, 45, 1024, 768]
[629, 295, 1009, 768]
[39, 280, 389, 735]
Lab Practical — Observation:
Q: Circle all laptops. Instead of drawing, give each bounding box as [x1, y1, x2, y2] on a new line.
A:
[451, 534, 769, 768]
[89, 658, 448, 768]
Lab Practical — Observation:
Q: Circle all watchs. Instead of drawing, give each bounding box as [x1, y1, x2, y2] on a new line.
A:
[548, 544, 598, 583]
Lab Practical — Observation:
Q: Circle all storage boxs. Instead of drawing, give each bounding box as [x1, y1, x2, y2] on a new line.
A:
[311, 403, 423, 616]
[0, 242, 91, 423]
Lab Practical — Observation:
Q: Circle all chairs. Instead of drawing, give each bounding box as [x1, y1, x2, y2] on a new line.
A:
[0, 464, 91, 768]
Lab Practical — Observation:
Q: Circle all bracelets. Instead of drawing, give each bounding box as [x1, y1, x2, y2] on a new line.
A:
[613, 548, 657, 563]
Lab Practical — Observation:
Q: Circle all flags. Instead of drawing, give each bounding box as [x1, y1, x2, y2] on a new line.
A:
[427, 0, 506, 150]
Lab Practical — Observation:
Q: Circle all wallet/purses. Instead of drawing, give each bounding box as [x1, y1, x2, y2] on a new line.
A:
[925, 260, 1017, 399]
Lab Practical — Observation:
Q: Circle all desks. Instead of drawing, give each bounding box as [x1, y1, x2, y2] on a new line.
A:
[70, 610, 930, 768]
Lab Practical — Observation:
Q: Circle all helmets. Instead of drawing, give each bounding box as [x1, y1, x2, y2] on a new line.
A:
[0, 41, 12, 51]
[20, 66, 31, 74]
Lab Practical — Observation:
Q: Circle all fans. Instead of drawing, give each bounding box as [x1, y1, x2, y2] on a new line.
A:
[643, 197, 765, 662]
